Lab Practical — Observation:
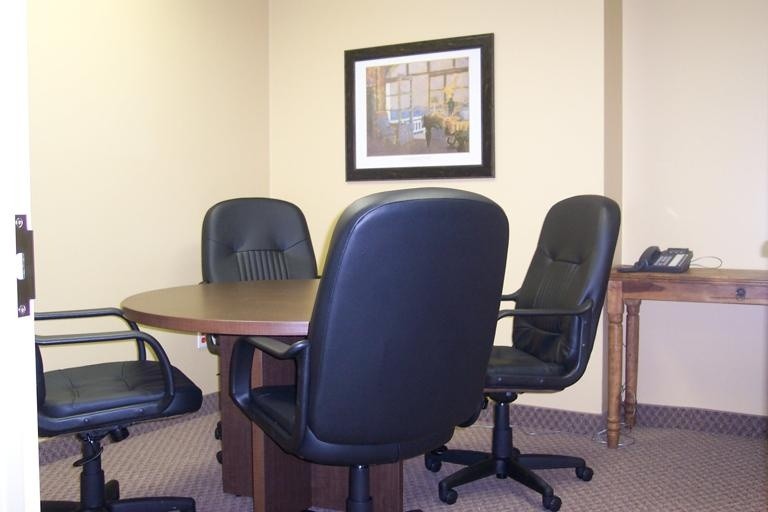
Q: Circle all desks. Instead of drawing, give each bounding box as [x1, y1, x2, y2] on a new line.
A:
[607, 267, 768, 450]
[120, 280, 402, 512]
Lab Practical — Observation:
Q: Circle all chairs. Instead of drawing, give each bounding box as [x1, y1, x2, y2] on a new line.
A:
[425, 194, 621, 511]
[228, 187, 509, 512]
[35, 307, 202, 512]
[199, 197, 321, 464]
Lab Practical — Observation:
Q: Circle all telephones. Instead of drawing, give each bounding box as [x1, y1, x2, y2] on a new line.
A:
[634, 246, 693, 273]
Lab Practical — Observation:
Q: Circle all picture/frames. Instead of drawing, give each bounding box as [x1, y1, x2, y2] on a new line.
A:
[344, 33, 495, 182]
